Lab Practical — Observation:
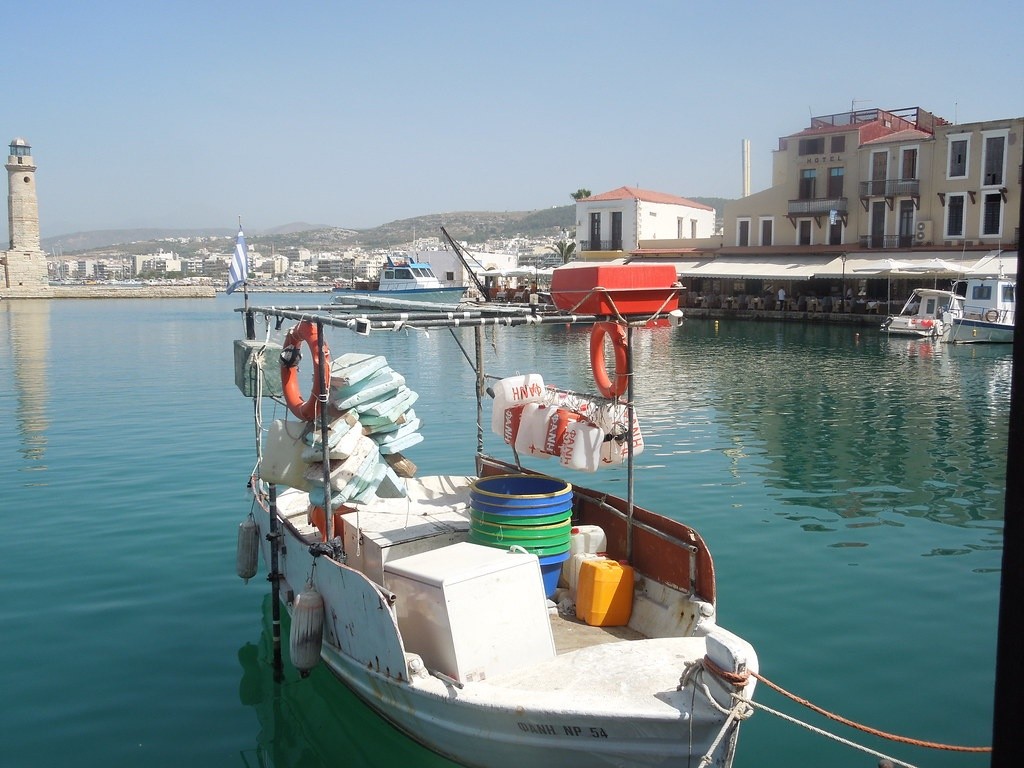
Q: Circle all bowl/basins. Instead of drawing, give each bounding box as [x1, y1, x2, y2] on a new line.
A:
[467, 474, 574, 599]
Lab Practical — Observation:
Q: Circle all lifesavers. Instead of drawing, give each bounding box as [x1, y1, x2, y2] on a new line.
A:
[279, 320, 331, 421]
[986, 309, 999, 322]
[589, 320, 629, 399]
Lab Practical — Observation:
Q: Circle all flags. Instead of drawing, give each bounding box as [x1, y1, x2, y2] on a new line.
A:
[226, 225, 250, 296]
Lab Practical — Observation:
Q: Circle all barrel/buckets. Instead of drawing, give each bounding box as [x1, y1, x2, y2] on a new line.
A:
[569, 552, 609, 613]
[491, 372, 645, 474]
[576, 559, 634, 626]
[562, 524, 608, 583]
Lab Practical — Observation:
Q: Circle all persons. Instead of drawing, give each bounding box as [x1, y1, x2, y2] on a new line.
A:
[777, 285, 786, 311]
[846, 287, 854, 299]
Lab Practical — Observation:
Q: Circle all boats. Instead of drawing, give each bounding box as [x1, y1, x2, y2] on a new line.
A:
[332, 255, 469, 305]
[941, 240, 1015, 344]
[232, 265, 760, 768]
[880, 238, 966, 337]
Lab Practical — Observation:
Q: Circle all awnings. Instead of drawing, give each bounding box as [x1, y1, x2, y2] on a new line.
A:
[814, 251, 1017, 316]
[558, 257, 624, 268]
[611, 256, 706, 280]
[681, 255, 839, 280]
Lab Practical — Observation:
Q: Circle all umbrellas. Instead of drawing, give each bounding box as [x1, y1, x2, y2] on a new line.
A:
[477, 266, 554, 292]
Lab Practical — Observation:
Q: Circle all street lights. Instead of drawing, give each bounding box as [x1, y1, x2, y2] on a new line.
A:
[840, 251, 847, 314]
